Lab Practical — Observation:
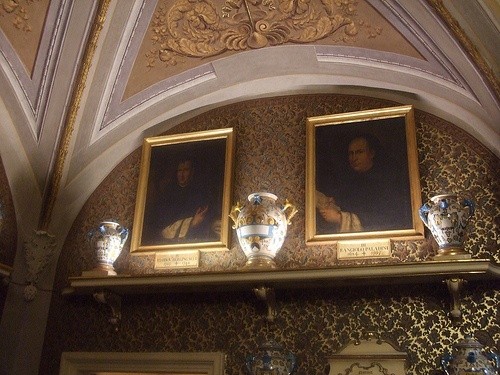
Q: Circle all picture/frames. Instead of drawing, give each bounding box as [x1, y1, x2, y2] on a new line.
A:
[60, 350, 227, 375]
[126, 127, 236, 257]
[305, 105, 425, 250]
